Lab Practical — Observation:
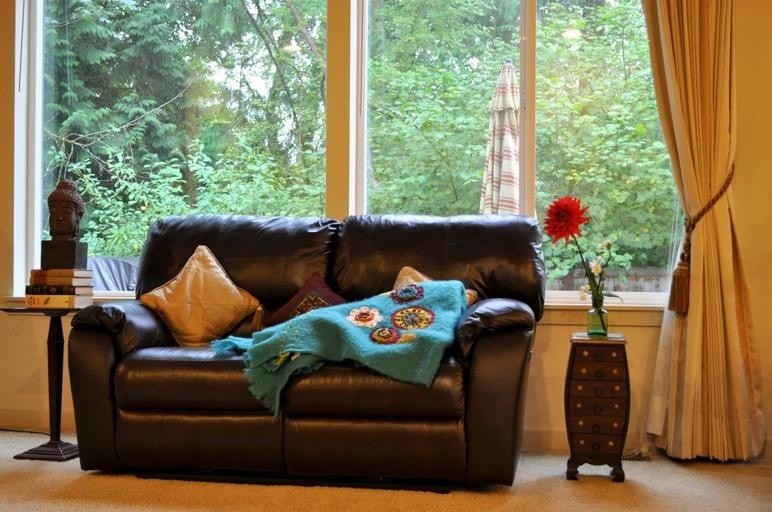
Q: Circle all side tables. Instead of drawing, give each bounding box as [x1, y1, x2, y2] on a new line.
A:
[1, 306, 83, 462]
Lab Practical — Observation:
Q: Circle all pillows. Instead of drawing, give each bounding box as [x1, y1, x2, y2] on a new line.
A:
[391, 266, 477, 306]
[139, 245, 260, 349]
[232, 304, 263, 337]
[264, 270, 347, 325]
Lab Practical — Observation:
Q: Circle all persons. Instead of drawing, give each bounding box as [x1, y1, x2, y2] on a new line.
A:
[47, 179, 85, 241]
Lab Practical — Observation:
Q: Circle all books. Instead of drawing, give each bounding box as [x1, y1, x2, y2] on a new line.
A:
[24, 269, 93, 309]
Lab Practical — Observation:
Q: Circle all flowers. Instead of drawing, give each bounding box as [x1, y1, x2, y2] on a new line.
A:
[545, 195, 624, 329]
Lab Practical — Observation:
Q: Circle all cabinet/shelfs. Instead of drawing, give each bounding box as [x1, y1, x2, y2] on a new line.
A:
[564, 334, 630, 483]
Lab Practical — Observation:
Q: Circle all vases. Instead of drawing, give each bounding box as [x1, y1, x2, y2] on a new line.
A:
[588, 297, 610, 337]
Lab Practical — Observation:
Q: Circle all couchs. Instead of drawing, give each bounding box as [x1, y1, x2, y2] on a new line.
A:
[66, 216, 544, 486]
